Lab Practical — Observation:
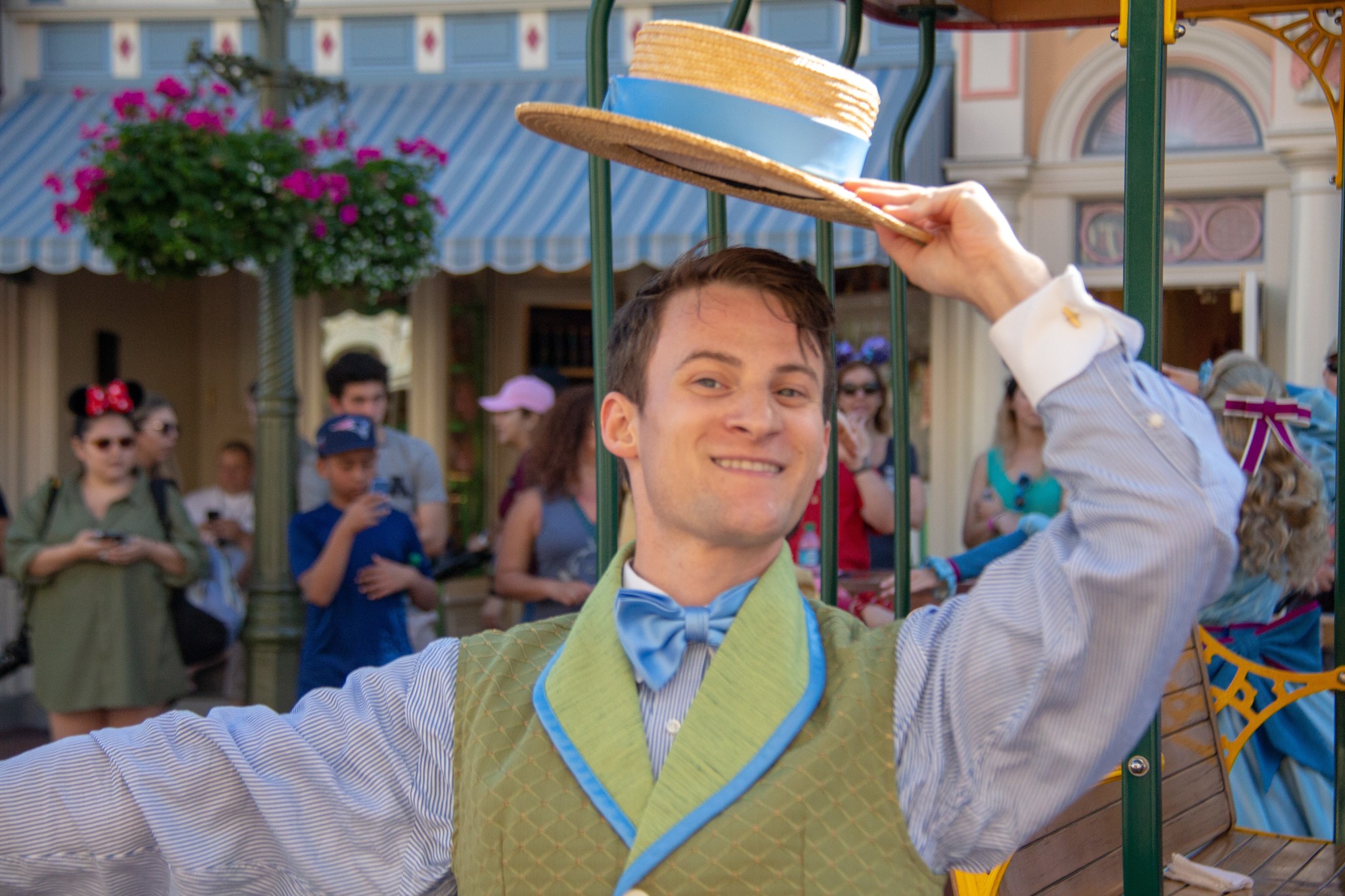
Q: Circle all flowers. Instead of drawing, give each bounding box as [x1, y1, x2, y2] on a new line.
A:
[44, 71, 451, 315]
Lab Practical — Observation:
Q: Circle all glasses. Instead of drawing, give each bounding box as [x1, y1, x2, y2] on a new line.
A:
[841, 381, 879, 394]
[1013, 474, 1031, 511]
[157, 420, 183, 436]
[1326, 362, 1338, 374]
[87, 436, 133, 450]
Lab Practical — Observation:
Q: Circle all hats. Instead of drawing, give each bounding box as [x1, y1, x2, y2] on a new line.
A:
[315, 413, 377, 459]
[478, 376, 555, 418]
[513, 19, 933, 249]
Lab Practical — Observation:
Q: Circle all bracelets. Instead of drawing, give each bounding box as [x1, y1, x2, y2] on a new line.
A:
[852, 456, 871, 476]
[988, 518, 1000, 538]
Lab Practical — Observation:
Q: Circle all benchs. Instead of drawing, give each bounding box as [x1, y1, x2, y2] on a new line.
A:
[946, 626, 1345, 894]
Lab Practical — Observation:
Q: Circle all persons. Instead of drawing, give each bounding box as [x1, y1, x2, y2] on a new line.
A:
[1159, 336, 1337, 612]
[2, 377, 214, 743]
[491, 386, 634, 623]
[129, 397, 179, 477]
[466, 372, 556, 631]
[0, 179, 1244, 896]
[0, 488, 13, 578]
[244, 373, 316, 500]
[179, 440, 255, 704]
[306, 349, 453, 654]
[881, 350, 1336, 842]
[789, 337, 932, 632]
[294, 414, 443, 713]
[962, 367, 1070, 551]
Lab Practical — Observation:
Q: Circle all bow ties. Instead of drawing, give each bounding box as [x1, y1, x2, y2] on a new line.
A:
[615, 578, 760, 691]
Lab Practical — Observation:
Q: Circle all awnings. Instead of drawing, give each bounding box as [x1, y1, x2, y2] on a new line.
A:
[0, 67, 958, 269]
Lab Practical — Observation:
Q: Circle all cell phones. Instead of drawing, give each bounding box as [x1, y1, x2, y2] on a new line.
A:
[208, 511, 220, 523]
[97, 530, 126, 542]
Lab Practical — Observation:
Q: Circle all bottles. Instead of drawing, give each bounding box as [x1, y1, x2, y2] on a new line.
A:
[399, 551, 423, 607]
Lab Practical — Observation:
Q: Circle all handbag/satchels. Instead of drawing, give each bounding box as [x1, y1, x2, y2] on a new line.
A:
[150, 468, 229, 666]
